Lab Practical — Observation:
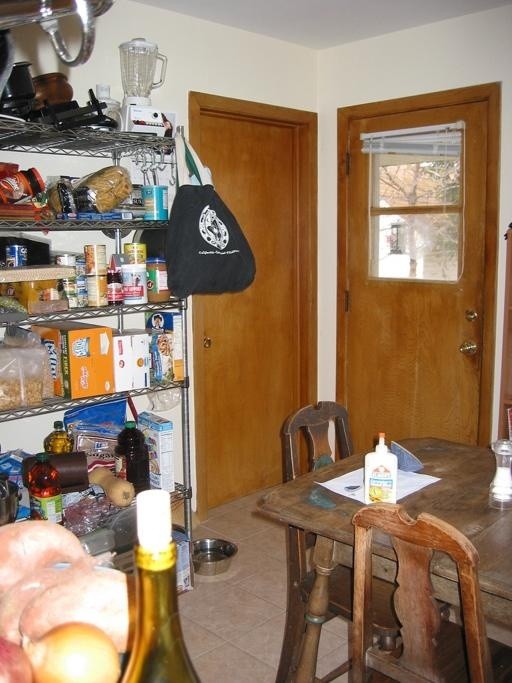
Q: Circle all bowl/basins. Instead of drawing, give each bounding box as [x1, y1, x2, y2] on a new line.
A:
[191, 535, 239, 575]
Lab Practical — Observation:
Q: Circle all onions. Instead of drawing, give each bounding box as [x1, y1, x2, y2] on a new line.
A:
[0, 623, 122, 683]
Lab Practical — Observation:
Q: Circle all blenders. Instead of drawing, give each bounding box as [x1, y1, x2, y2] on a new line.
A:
[118, 36, 168, 139]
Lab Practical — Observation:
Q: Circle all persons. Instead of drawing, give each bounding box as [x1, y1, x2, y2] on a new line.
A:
[153, 314, 163, 329]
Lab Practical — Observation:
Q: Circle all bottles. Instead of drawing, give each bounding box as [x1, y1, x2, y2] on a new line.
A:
[119, 487, 201, 683]
[28, 451, 65, 528]
[44, 421, 74, 453]
[114, 419, 150, 496]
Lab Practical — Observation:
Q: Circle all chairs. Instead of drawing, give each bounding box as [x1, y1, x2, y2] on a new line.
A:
[281, 400, 512, 681]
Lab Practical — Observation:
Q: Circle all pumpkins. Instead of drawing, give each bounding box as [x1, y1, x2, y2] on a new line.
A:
[88, 467, 135, 507]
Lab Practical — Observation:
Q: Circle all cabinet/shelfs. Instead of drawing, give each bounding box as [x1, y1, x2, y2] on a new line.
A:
[0, 120, 195, 591]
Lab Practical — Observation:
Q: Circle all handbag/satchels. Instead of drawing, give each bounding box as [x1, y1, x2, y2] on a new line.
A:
[166, 186, 256, 299]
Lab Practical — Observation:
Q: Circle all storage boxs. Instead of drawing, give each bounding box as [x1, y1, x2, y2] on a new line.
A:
[30, 320, 150, 399]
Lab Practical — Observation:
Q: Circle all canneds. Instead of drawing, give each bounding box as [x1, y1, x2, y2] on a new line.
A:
[56, 242, 171, 309]
[0, 162, 46, 205]
[5, 245, 29, 269]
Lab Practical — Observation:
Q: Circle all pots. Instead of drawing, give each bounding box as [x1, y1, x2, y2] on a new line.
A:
[2, 60, 73, 107]
